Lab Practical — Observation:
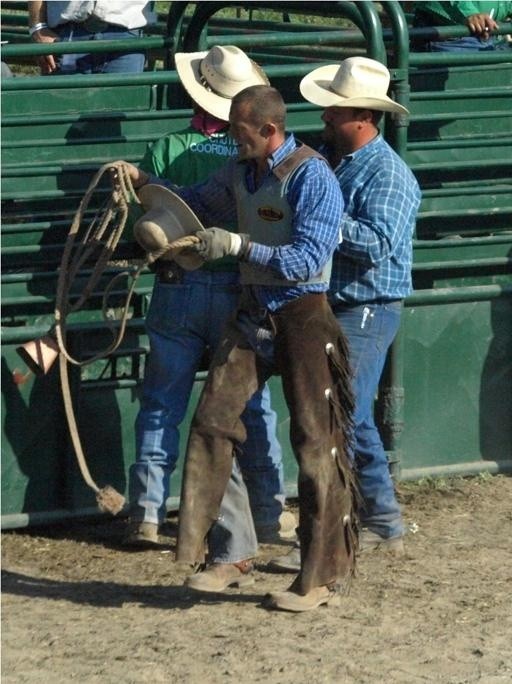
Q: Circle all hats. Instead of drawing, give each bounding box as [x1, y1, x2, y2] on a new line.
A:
[134, 184, 207, 272]
[174, 45, 270, 122]
[300, 57, 410, 115]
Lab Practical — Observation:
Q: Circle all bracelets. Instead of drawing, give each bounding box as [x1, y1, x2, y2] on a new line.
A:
[29, 22, 47, 35]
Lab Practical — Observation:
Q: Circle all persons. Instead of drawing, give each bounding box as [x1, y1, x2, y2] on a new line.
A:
[414, 0, 512, 52]
[111, 82, 361, 614]
[112, 41, 296, 546]
[27, 1, 157, 76]
[267, 55, 424, 572]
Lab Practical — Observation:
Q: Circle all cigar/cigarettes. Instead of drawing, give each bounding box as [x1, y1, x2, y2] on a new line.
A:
[485, 8, 495, 31]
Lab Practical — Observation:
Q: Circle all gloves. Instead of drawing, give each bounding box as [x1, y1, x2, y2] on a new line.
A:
[190, 225, 250, 266]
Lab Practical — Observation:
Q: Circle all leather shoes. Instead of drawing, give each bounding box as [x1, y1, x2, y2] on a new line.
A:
[264, 585, 341, 611]
[360, 527, 402, 543]
[184, 558, 255, 593]
[123, 522, 159, 547]
[254, 509, 295, 542]
